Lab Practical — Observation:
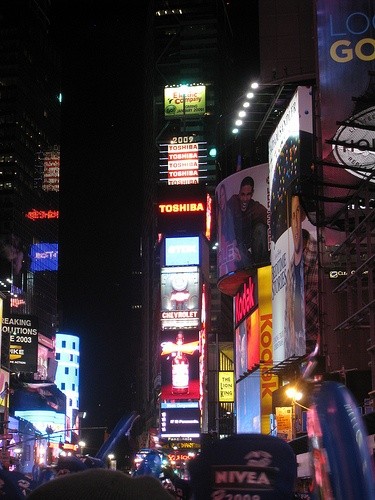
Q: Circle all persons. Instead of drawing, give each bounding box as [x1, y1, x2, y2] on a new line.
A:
[284, 193, 320, 359]
[215, 175, 267, 276]
